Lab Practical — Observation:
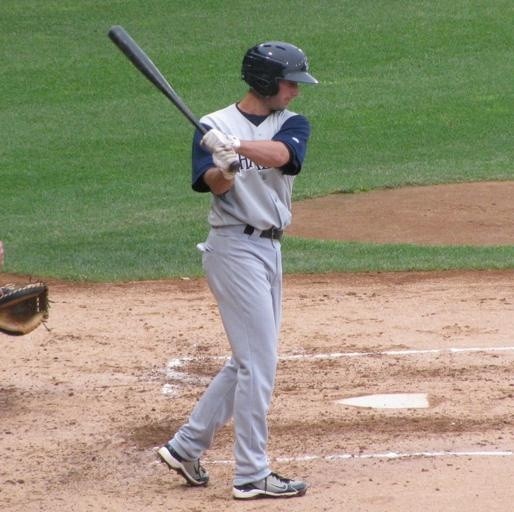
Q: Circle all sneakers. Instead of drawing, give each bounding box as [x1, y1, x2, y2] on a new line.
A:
[233, 474, 307, 500]
[157, 444, 209, 486]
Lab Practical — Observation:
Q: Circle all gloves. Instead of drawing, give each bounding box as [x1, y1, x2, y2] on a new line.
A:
[200, 130, 241, 180]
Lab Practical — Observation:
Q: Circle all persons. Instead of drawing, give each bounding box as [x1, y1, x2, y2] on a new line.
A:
[156, 41, 321, 500]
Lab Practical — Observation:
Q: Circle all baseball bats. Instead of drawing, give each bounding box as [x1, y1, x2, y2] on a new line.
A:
[105, 25, 239, 173]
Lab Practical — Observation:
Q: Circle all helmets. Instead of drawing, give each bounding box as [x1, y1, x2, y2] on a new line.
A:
[241, 41, 319, 99]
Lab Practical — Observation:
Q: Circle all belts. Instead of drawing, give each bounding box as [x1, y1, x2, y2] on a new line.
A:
[244, 225, 283, 238]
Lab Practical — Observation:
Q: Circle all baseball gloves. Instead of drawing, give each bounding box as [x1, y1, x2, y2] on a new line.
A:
[0, 271, 48, 334]
